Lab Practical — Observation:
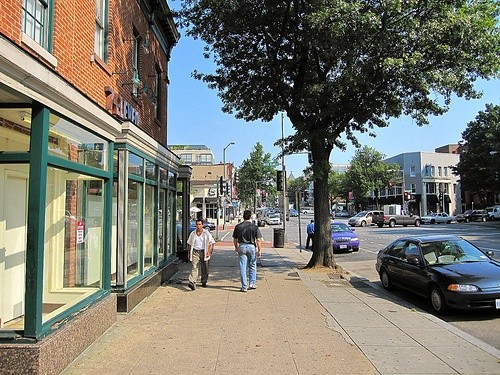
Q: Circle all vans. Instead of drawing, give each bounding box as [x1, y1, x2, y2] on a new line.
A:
[348, 210, 385, 227]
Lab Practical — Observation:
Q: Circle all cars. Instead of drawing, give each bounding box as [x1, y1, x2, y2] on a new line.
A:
[303, 207, 314, 215]
[420, 213, 454, 224]
[376, 235, 500, 316]
[335, 211, 350, 217]
[190, 220, 215, 232]
[330, 223, 360, 254]
[176, 222, 197, 240]
[255, 208, 297, 225]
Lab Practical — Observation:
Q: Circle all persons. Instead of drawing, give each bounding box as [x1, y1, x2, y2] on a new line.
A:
[187, 219, 215, 291]
[232, 210, 262, 291]
[251, 212, 256, 225]
[305, 219, 314, 250]
[238, 213, 243, 223]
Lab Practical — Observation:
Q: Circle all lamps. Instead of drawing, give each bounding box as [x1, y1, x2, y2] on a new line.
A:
[111, 66, 137, 76]
[148, 70, 171, 84]
[122, 32, 151, 49]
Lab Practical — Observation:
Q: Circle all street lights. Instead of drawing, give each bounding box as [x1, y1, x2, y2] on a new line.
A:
[223, 142, 235, 230]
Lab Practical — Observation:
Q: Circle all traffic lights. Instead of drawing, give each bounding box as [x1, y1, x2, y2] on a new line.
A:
[388, 183, 394, 188]
[404, 192, 408, 201]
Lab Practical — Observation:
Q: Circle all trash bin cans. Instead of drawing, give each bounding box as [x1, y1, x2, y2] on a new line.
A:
[273, 228, 285, 248]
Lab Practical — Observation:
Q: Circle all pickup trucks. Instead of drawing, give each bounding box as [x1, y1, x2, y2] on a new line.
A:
[372, 210, 422, 228]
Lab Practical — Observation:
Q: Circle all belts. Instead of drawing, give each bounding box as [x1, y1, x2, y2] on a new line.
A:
[196, 250, 203, 252]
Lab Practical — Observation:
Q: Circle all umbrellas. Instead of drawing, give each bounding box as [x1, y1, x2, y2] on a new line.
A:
[190, 206, 202, 212]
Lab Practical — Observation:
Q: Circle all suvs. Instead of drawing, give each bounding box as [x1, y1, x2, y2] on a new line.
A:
[456, 209, 490, 223]
[485, 205, 500, 221]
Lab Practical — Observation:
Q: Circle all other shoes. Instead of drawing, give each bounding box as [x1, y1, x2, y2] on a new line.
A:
[202, 283, 207, 287]
[249, 284, 258, 288]
[188, 283, 196, 290]
[242, 288, 247, 291]
